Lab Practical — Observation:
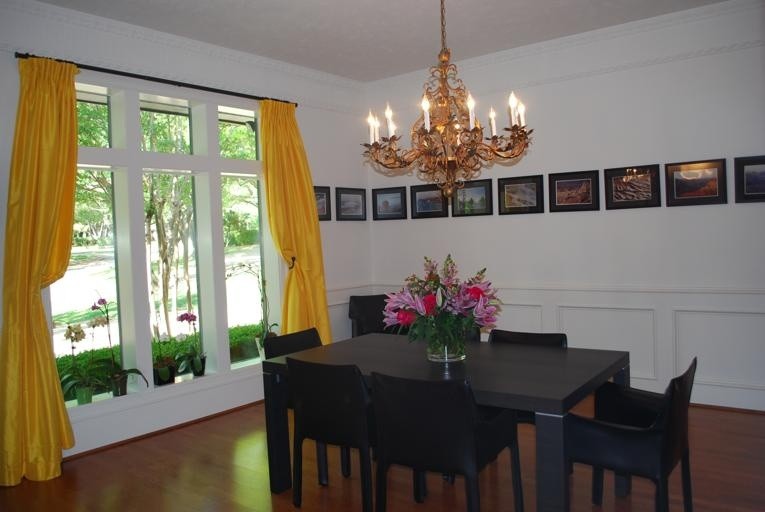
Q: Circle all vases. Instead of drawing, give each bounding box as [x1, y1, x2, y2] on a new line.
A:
[75, 386, 92, 405]
[255, 337, 279, 361]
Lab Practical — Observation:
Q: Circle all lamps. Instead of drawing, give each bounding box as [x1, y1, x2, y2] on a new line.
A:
[359, 0, 533, 198]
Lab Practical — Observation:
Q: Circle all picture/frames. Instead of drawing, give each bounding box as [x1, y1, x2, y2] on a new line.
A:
[604, 164, 661, 209]
[314, 186, 331, 221]
[548, 170, 600, 212]
[734, 155, 765, 202]
[497, 174, 544, 215]
[665, 159, 728, 206]
[372, 187, 407, 220]
[411, 183, 449, 219]
[336, 187, 367, 221]
[451, 179, 492, 217]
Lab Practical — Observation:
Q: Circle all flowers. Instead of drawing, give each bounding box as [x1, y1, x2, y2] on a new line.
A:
[382, 253, 504, 358]
[225, 262, 279, 350]
[60, 298, 207, 395]
